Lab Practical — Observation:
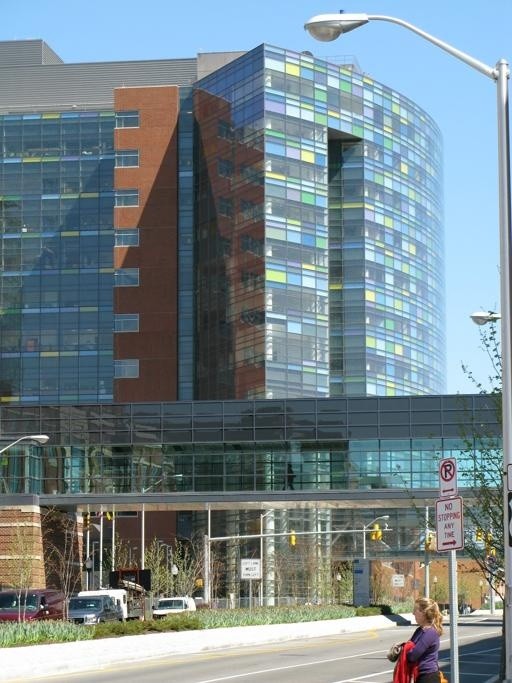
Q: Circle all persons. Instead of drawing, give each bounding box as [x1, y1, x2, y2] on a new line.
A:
[396, 597, 446, 682]
[282, 463, 296, 490]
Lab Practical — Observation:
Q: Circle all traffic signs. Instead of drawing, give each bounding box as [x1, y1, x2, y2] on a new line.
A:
[436, 496, 464, 554]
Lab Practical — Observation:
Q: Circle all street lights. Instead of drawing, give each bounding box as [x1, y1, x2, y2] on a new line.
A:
[0, 430, 52, 456]
[138, 473, 186, 571]
[363, 514, 390, 559]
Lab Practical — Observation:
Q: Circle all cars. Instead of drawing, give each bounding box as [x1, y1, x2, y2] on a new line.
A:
[0, 587, 196, 627]
